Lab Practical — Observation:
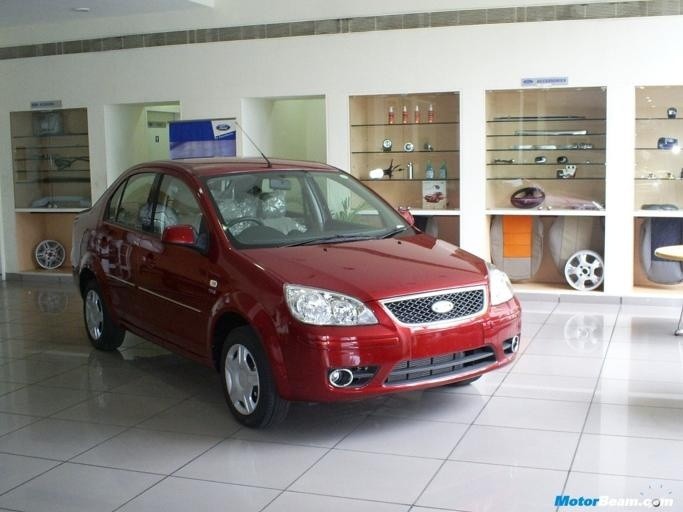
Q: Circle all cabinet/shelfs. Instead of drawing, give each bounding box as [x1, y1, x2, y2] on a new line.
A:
[14, 130, 92, 215]
[348, 115, 683, 291]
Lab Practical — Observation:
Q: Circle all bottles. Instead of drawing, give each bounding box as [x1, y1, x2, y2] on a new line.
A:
[427, 104, 434, 124]
[414, 105, 421, 124]
[388, 105, 394, 125]
[438, 161, 448, 179]
[402, 104, 408, 125]
[407, 160, 414, 180]
[423, 160, 435, 180]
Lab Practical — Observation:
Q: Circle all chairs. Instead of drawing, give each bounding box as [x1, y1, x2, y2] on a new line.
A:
[216, 195, 308, 237]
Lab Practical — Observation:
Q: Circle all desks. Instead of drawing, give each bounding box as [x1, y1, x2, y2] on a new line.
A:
[650, 243, 683, 334]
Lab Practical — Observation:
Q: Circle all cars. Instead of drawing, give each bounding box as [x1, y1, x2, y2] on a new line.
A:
[70, 158, 522, 430]
